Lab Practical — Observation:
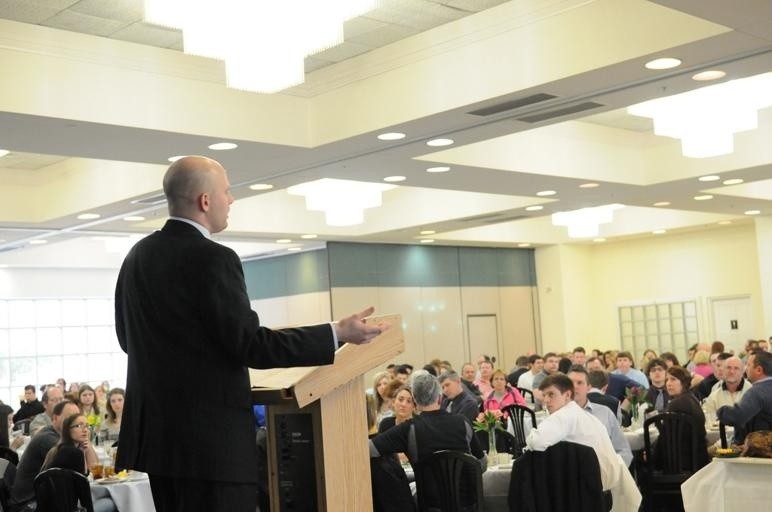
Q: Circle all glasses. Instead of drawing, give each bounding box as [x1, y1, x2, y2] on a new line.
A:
[69, 424, 93, 428]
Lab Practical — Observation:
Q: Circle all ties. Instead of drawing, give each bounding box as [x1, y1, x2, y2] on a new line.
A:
[653, 389, 662, 409]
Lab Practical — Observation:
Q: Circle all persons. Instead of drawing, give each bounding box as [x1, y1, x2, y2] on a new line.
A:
[365, 338, 771, 512]
[0, 377, 127, 511]
[114, 158, 393, 510]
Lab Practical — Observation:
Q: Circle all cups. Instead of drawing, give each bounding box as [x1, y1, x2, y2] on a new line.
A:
[84, 426, 149, 483]
[480, 449, 514, 468]
[12, 430, 23, 440]
[525, 402, 536, 416]
